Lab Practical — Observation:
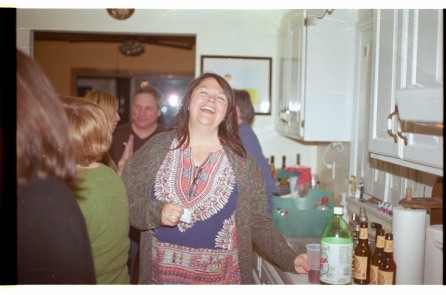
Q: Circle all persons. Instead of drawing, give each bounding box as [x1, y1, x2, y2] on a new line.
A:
[60, 96, 129, 285]
[121, 73, 311, 285]
[233, 89, 274, 218]
[17, 47, 97, 285]
[89, 85, 165, 284]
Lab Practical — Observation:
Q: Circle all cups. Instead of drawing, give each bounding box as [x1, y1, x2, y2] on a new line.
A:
[306, 243, 320, 283]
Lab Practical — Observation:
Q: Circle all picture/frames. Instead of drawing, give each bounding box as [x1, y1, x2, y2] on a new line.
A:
[201, 55, 272, 115]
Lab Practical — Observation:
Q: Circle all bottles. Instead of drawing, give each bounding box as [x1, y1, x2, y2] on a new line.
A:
[296, 154, 302, 169]
[352, 225, 359, 270]
[279, 156, 288, 186]
[269, 156, 276, 178]
[370, 230, 385, 285]
[378, 233, 395, 285]
[315, 197, 329, 211]
[319, 207, 352, 285]
[359, 207, 368, 223]
[354, 223, 370, 285]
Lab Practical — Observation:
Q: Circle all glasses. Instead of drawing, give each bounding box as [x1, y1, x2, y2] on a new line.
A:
[189, 166, 202, 197]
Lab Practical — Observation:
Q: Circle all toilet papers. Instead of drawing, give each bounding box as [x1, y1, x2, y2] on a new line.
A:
[392, 207, 426, 285]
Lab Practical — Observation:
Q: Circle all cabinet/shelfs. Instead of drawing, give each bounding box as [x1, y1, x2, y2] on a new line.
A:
[277, 9, 360, 143]
[252, 250, 285, 285]
[367, 9, 444, 176]
